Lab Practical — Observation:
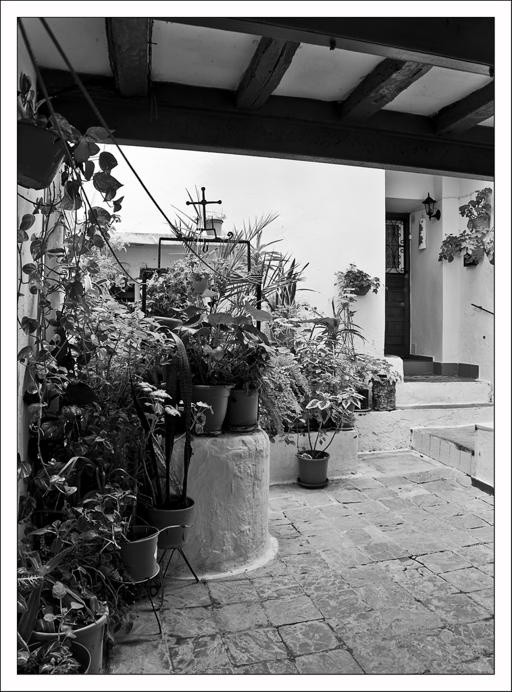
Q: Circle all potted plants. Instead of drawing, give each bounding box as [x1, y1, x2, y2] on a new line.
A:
[17, 357, 199, 675]
[334, 263, 379, 301]
[19, 74, 125, 212]
[175, 300, 273, 434]
[292, 378, 366, 486]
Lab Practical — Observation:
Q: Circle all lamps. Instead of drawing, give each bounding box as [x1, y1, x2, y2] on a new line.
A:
[422, 192, 441, 221]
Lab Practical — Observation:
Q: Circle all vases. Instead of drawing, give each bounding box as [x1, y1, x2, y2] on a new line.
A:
[206, 218, 223, 236]
